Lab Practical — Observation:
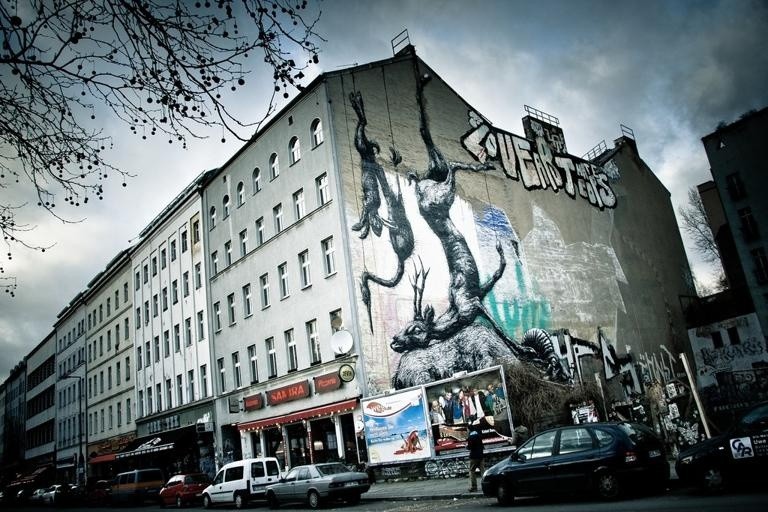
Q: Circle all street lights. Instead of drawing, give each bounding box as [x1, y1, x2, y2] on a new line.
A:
[57, 368, 84, 485]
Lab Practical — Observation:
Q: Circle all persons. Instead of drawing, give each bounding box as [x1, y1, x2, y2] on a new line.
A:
[429, 383, 508, 426]
[463, 424, 486, 492]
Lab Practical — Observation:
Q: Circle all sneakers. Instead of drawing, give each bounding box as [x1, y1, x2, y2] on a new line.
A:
[467, 486, 478, 492]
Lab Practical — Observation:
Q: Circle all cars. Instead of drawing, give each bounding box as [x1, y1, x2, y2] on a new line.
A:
[482, 402, 768, 506]
